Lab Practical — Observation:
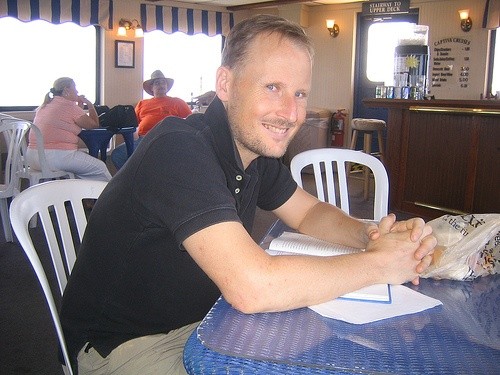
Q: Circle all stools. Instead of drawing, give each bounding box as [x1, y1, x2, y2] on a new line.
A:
[346, 119, 387, 199]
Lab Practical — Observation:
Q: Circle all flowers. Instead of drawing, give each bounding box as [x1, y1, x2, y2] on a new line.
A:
[404, 54, 418, 86]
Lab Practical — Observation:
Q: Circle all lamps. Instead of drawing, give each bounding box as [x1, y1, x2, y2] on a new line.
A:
[327, 20, 339, 38]
[458, 10, 472, 32]
[118, 18, 144, 39]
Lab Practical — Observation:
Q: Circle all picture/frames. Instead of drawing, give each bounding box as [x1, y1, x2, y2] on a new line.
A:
[116, 40, 135, 69]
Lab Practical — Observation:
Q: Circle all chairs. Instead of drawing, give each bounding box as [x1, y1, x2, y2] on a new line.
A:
[0, 111, 107, 375]
[290, 148, 389, 221]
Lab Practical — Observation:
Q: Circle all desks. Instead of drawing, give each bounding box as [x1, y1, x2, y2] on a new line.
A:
[183, 216, 500, 375]
[77, 127, 136, 166]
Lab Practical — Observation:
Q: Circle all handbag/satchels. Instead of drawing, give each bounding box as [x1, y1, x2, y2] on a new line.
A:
[418, 213, 500, 282]
[94, 105, 138, 128]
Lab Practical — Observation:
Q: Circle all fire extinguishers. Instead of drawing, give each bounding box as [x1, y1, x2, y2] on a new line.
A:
[330, 109, 345, 146]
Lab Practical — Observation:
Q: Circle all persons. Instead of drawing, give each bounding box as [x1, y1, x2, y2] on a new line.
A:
[111, 70, 192, 171]
[26, 77, 113, 182]
[58, 14, 437, 375]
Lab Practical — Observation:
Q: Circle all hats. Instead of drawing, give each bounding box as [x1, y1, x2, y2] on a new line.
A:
[143, 70, 174, 96]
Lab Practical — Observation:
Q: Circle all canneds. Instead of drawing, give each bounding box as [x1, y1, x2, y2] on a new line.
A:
[375, 85, 420, 100]
